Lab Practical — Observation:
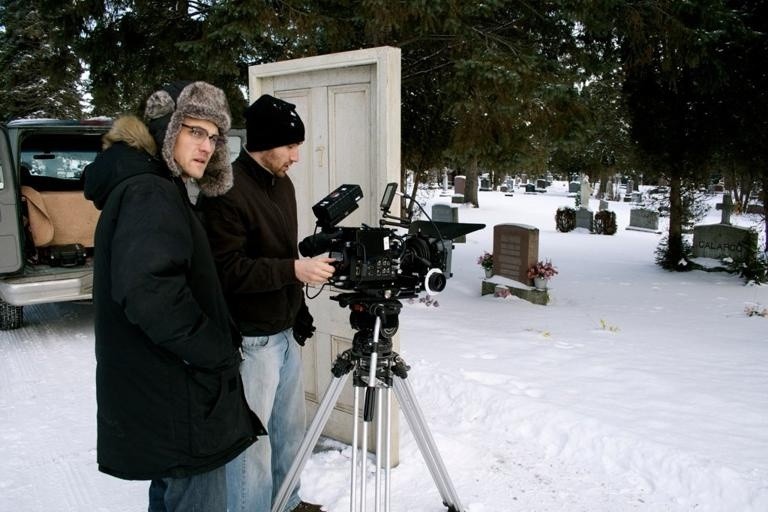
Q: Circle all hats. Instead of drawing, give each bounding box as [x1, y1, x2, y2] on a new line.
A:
[241, 93, 305, 153]
[143, 75, 234, 198]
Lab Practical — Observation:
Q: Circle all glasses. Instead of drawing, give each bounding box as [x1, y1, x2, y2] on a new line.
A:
[181, 122, 225, 149]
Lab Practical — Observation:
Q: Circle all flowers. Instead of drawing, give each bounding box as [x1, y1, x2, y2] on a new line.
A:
[526, 259, 557, 280]
[478, 252, 494, 271]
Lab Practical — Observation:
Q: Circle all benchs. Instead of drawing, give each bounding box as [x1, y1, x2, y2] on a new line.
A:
[28, 188, 105, 260]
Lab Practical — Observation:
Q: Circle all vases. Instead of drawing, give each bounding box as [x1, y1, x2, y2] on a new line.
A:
[534, 276, 548, 291]
[484, 268, 493, 279]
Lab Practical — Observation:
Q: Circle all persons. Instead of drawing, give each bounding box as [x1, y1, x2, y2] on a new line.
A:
[80, 80, 269, 511]
[192, 94, 337, 512]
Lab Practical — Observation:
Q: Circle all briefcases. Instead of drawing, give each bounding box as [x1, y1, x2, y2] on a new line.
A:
[47, 243, 87, 269]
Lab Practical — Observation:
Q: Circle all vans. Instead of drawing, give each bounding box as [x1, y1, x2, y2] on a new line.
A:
[0, 117, 120, 332]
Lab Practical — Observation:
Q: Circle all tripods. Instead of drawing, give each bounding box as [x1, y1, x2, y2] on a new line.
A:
[271, 334, 466, 512]
